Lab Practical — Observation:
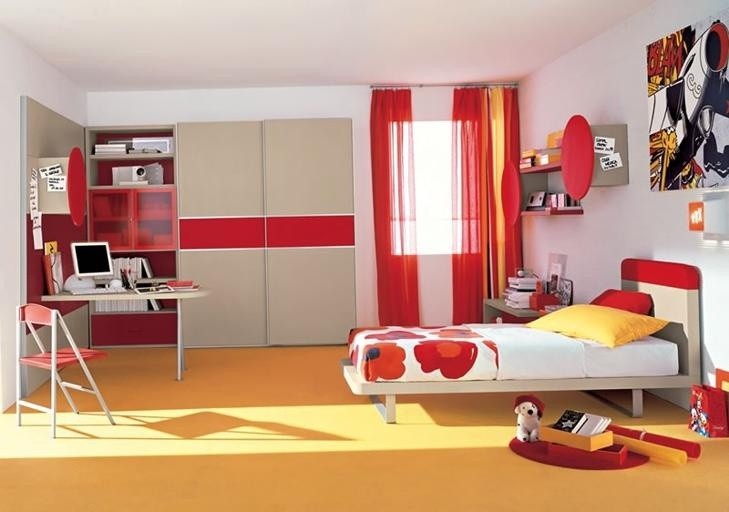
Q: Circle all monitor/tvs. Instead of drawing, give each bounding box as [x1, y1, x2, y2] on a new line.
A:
[71, 242, 113, 289]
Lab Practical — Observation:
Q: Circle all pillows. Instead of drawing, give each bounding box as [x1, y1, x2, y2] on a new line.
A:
[522, 304, 670, 349]
[589, 289, 652, 315]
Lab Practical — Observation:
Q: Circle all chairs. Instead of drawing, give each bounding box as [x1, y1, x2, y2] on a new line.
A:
[16, 303, 116, 439]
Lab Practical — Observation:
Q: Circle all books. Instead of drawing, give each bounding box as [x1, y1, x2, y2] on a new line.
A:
[166, 279, 194, 289]
[92, 256, 160, 313]
[543, 304, 568, 312]
[174, 284, 202, 292]
[128, 149, 157, 154]
[92, 143, 128, 155]
[500, 275, 543, 311]
[118, 179, 149, 186]
[551, 408, 613, 437]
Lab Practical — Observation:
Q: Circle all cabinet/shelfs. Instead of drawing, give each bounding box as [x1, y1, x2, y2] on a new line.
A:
[520, 161, 583, 216]
[178, 118, 356, 349]
[85, 124, 178, 350]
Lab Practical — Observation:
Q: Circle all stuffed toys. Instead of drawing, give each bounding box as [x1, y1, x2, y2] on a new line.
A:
[512, 394, 546, 443]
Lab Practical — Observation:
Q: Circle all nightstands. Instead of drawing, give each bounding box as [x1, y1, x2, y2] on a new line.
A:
[483, 298, 541, 324]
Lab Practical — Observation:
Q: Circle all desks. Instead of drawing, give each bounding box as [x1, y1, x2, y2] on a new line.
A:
[41, 286, 208, 381]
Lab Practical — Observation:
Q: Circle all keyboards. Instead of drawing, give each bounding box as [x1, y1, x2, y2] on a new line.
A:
[70, 286, 128, 295]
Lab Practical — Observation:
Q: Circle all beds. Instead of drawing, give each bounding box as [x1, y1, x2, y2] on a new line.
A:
[340, 257, 701, 423]
